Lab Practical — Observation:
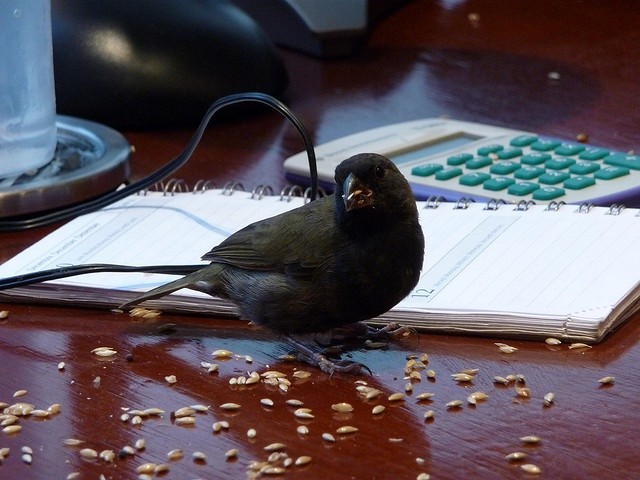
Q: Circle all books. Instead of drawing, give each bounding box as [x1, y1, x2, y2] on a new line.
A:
[0, 182, 640, 344]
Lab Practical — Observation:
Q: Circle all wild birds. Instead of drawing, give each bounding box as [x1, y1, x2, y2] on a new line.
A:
[118, 152, 425, 380]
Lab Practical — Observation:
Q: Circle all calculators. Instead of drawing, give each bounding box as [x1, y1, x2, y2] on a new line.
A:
[283, 118, 640, 207]
